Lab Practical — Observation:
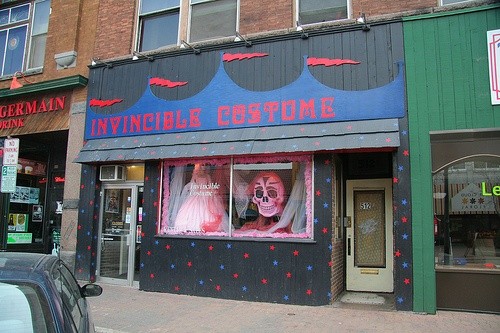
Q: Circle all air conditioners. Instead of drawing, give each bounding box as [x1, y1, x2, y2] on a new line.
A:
[99, 165, 125, 182]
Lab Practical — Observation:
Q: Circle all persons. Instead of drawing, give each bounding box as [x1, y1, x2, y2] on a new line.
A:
[174, 163, 236, 232]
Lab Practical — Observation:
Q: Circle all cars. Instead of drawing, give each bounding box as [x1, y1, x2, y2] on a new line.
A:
[0, 251, 103, 333]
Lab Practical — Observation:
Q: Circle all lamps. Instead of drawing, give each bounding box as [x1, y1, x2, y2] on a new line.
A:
[296, 21, 308, 38]
[179, 40, 200, 54]
[91, 57, 111, 68]
[10, 71, 32, 89]
[357, 12, 371, 31]
[234, 32, 252, 46]
[132, 51, 153, 61]
